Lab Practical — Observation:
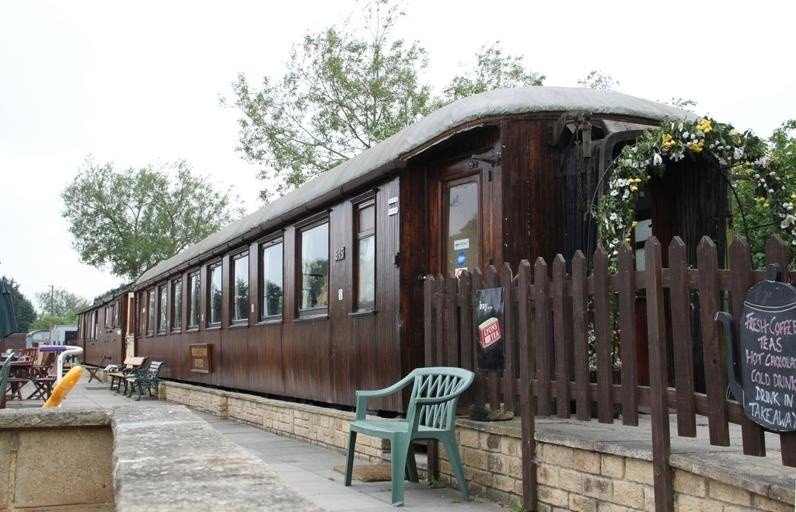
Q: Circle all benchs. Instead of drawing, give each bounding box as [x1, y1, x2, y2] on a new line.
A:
[107, 356, 164, 402]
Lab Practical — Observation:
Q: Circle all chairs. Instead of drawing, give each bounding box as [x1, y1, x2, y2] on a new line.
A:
[85, 354, 107, 383]
[7, 347, 57, 403]
[343, 366, 477, 508]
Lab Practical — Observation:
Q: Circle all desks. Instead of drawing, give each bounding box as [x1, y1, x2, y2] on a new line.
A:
[0, 353, 32, 400]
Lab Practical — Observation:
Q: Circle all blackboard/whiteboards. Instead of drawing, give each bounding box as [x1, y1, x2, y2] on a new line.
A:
[743, 280, 796, 432]
